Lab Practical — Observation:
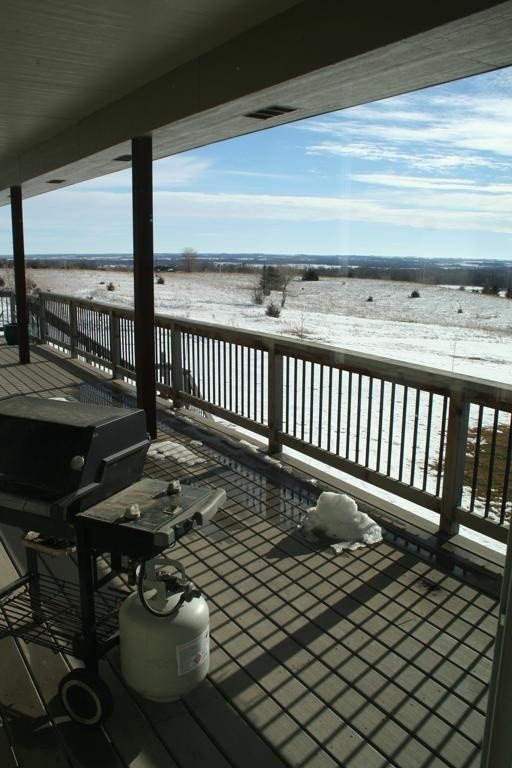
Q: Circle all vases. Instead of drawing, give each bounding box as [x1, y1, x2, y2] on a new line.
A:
[2, 322, 21, 346]
